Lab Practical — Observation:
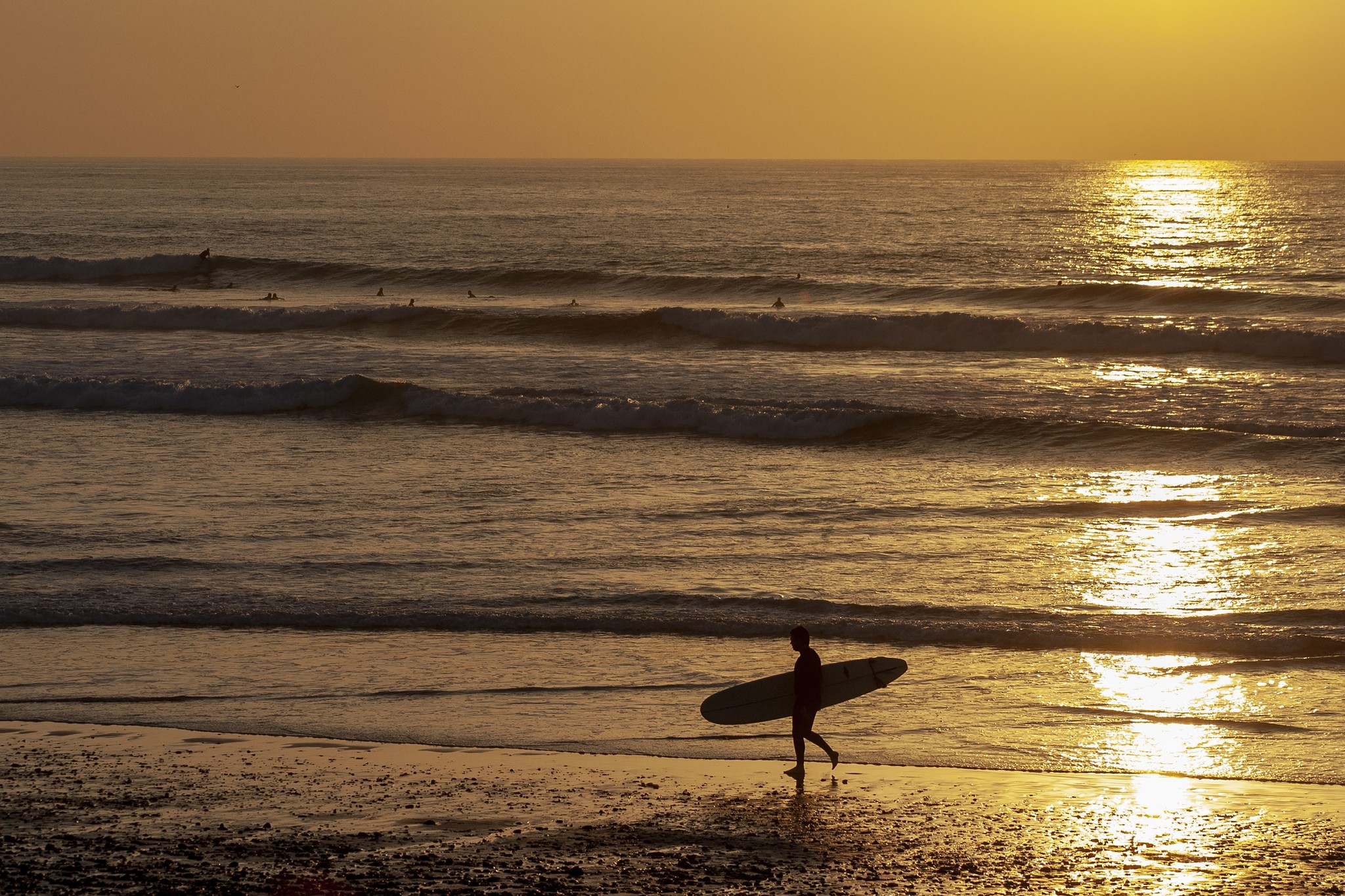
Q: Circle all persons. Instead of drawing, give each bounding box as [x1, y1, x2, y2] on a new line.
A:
[199, 248, 210, 263]
[376, 288, 384, 296]
[796, 273, 801, 279]
[468, 290, 476, 298]
[771, 297, 785, 307]
[569, 299, 578, 306]
[783, 625, 839, 773]
[271, 293, 278, 300]
[409, 298, 414, 307]
[265, 293, 272, 300]
[1057, 281, 1062, 286]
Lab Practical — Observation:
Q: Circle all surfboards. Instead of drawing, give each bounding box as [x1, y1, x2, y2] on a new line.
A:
[700, 656, 909, 726]
